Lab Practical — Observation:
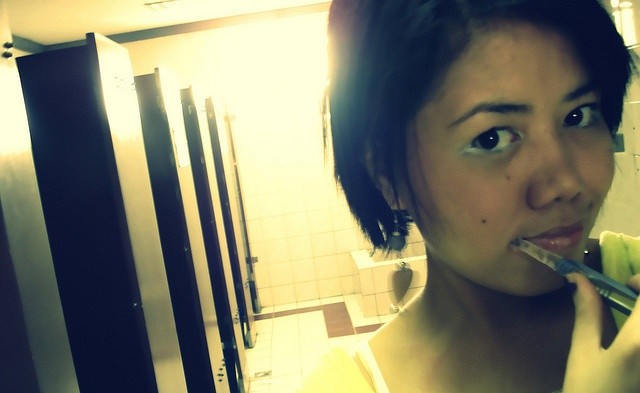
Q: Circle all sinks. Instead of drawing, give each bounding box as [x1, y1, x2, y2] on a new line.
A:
[349, 248, 427, 316]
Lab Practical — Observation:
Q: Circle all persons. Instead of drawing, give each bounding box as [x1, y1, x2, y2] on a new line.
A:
[270, 0, 639, 393]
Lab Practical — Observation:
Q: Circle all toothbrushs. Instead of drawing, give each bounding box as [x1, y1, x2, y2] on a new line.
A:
[511, 236, 639, 318]
